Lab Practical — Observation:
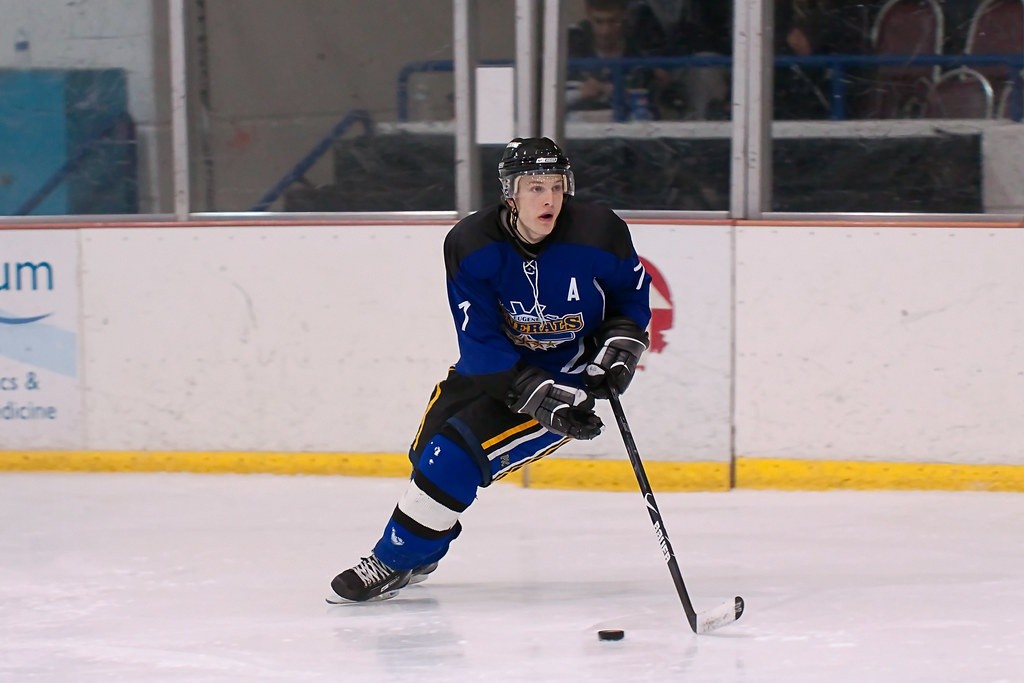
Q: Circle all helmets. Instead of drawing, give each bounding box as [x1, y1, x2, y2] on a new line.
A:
[499, 137, 571, 193]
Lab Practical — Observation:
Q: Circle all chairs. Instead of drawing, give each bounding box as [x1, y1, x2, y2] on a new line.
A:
[861, 0, 1024, 121]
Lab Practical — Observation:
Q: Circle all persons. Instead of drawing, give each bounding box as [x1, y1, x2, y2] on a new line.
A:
[284, 155, 440, 212]
[569, 1, 875, 119]
[325, 136, 652, 607]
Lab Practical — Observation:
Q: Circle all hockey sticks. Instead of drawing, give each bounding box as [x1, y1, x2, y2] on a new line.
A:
[605, 386, 745, 635]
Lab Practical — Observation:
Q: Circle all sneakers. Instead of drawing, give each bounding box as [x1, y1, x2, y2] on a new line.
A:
[408, 562, 438, 584]
[325, 553, 412, 607]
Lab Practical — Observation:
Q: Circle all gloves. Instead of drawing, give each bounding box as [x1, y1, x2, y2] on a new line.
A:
[584, 319, 650, 399]
[504, 366, 603, 440]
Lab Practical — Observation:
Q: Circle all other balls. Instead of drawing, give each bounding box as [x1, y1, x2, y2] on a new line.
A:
[598, 630, 624, 641]
